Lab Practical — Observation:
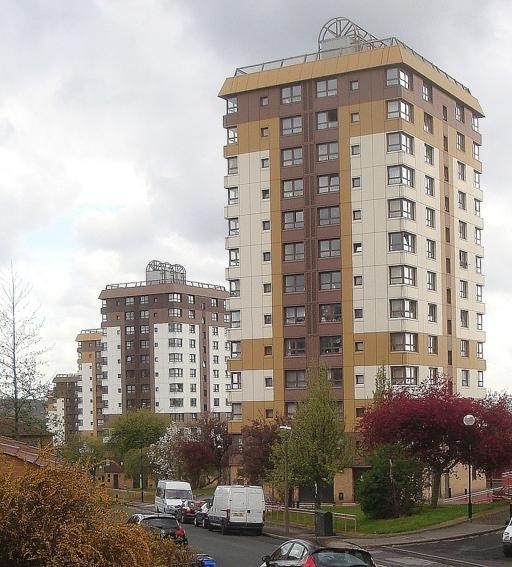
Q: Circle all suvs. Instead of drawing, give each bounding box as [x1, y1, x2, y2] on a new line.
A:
[175, 498, 205, 522]
[122, 506, 191, 552]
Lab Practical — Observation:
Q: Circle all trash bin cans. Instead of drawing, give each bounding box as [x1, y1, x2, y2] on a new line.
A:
[314, 511, 333, 536]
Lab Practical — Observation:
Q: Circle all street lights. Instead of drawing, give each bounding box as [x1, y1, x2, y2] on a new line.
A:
[134, 440, 145, 502]
[279, 426, 292, 532]
[462, 414, 476, 518]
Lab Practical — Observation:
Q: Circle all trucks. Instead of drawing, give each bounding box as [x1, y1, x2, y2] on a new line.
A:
[206, 484, 266, 534]
[154, 479, 194, 517]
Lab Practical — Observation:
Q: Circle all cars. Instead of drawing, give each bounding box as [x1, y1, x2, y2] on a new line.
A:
[257, 536, 379, 566]
[500, 512, 512, 551]
[194, 501, 213, 526]
